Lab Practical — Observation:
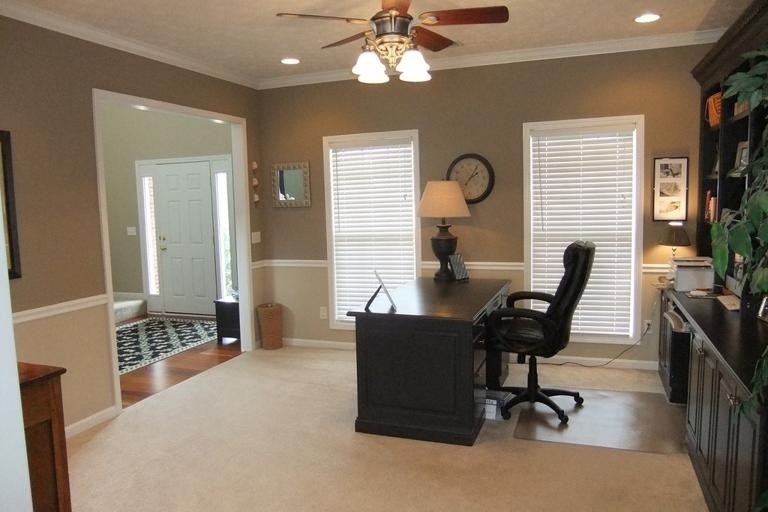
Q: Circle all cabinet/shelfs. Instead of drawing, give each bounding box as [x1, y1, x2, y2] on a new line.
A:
[17, 361, 71, 511]
[658, 286, 694, 403]
[689, 1, 768, 299]
[712, 373, 756, 511]
[687, 332, 715, 465]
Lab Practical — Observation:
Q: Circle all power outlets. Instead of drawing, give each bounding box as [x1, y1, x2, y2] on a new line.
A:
[645, 319, 652, 333]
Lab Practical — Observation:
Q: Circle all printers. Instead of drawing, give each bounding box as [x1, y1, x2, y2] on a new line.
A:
[672, 256, 715, 293]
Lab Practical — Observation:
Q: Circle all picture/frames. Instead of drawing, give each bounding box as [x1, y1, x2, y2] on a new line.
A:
[0, 131, 22, 279]
[271, 160, 312, 208]
[652, 157, 688, 222]
[734, 141, 748, 171]
[365, 271, 397, 312]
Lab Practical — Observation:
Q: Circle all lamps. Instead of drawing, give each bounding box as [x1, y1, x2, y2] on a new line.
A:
[415, 181, 471, 278]
[351, 1, 432, 86]
[656, 225, 691, 276]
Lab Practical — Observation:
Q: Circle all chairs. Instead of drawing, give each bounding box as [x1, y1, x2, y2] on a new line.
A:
[487, 239, 596, 424]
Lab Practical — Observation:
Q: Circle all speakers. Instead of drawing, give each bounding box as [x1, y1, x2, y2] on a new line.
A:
[742, 295, 762, 316]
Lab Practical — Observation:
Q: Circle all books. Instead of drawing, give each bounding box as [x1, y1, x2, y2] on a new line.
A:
[704, 188, 717, 222]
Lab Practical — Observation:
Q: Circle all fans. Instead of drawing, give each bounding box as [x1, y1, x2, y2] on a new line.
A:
[275, 1, 509, 53]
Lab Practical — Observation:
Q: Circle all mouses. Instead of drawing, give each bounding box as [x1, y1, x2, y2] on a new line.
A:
[690, 290, 707, 296]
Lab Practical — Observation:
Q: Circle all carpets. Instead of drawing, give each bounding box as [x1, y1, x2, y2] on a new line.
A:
[115, 316, 217, 375]
[513, 384, 689, 455]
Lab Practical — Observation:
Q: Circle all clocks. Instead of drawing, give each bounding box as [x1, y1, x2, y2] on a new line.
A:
[446, 153, 494, 204]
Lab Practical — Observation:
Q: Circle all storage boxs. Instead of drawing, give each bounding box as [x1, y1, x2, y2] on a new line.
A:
[474, 386, 512, 420]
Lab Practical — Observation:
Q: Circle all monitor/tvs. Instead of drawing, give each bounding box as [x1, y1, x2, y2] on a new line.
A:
[726, 245, 748, 299]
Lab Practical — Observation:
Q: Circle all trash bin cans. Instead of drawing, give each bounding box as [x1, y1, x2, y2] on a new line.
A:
[257, 303, 284, 350]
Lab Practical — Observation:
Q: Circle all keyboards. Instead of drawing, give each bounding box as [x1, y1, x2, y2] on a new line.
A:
[716, 294, 741, 311]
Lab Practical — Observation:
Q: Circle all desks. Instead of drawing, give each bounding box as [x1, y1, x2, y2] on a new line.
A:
[346, 277, 510, 446]
